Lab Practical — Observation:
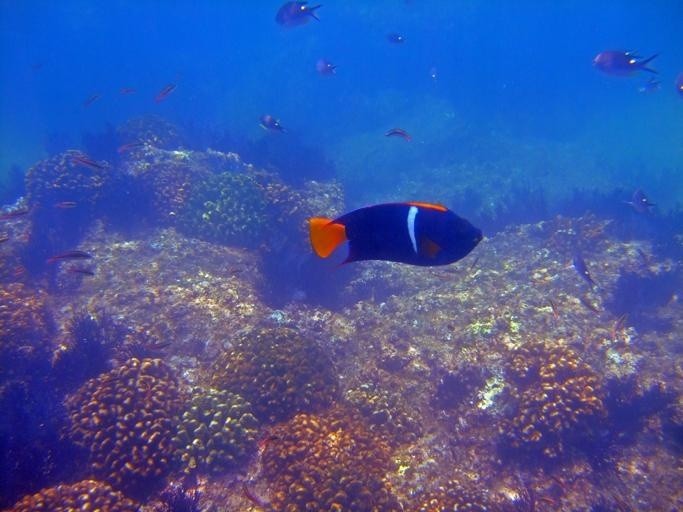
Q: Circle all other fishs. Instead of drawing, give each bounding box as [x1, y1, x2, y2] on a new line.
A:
[571, 252, 601, 292]
[386, 128, 411, 140]
[307, 200, 485, 267]
[622, 189, 658, 215]
[0, 82, 176, 277]
[259, 113, 287, 134]
[590, 49, 659, 79]
[388, 32, 406, 43]
[273, 0, 331, 27]
[315, 59, 337, 76]
